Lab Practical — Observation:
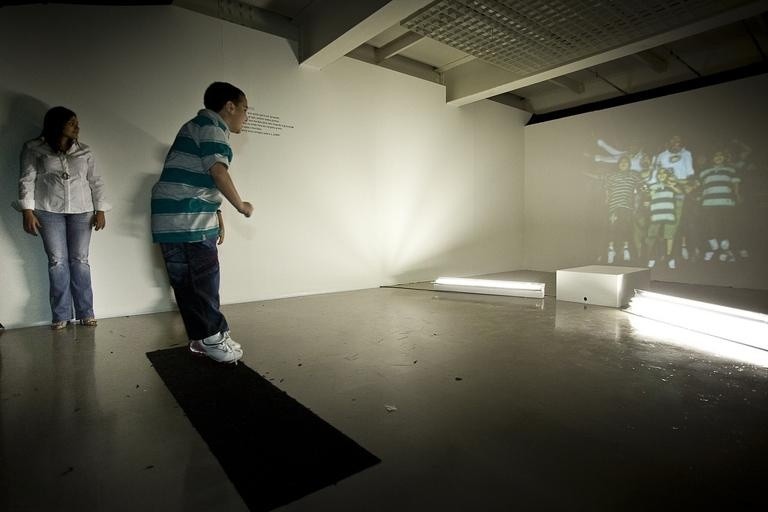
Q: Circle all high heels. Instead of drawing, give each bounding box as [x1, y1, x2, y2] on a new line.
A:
[80, 318, 97, 327]
[53, 320, 71, 331]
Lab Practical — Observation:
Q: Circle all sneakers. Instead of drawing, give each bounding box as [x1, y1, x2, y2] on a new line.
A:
[190, 330, 244, 364]
[597, 246, 748, 270]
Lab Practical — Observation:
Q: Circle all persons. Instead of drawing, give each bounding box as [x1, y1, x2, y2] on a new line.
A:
[150, 80, 258, 363]
[9, 105, 108, 330]
[581, 128, 761, 274]
